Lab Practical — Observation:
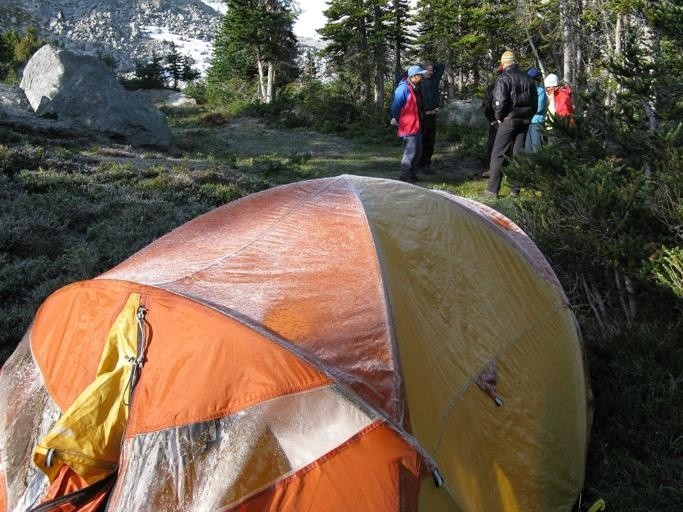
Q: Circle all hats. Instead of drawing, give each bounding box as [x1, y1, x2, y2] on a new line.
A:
[501, 51, 515, 66]
[528, 68, 540, 77]
[543, 73, 559, 88]
[408, 66, 426, 76]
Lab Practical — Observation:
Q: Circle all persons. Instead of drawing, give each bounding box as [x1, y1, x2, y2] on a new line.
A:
[482, 63, 614, 178]
[390, 66, 428, 184]
[416, 61, 446, 176]
[478, 49, 538, 199]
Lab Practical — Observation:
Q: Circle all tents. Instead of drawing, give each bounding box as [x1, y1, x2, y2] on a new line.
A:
[2, 172, 609, 511]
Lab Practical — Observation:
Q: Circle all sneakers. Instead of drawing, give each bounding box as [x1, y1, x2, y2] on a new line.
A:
[423, 166, 434, 175]
[401, 175, 418, 183]
[478, 191, 497, 199]
